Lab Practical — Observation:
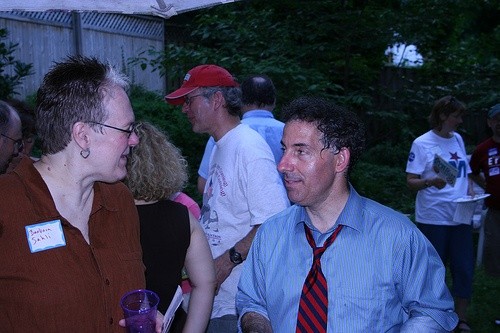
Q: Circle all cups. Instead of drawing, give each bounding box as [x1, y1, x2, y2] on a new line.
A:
[120, 289, 159, 333]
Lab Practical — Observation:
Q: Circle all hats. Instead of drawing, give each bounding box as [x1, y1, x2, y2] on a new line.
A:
[487, 104, 500, 119]
[164, 65, 239, 105]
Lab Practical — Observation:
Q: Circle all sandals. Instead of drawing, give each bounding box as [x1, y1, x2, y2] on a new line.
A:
[454, 320, 468, 333]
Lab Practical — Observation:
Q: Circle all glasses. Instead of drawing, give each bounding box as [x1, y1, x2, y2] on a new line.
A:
[184, 94, 207, 104]
[84, 121, 141, 139]
[0, 133, 24, 149]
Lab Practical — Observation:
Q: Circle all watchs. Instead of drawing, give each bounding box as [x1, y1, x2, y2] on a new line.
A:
[229, 248, 243, 264]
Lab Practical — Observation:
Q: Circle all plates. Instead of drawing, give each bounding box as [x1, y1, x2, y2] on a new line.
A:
[453, 194, 491, 204]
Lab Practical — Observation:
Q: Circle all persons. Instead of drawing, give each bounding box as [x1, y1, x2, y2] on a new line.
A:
[234, 97, 459, 333]
[467, 103, 500, 277]
[0, 54, 165, 333]
[120, 122, 217, 333]
[197, 75, 286, 194]
[405, 98, 473, 333]
[0, 101, 25, 174]
[165, 65, 290, 333]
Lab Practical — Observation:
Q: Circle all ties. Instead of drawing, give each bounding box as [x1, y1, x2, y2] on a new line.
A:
[295, 221, 344, 333]
